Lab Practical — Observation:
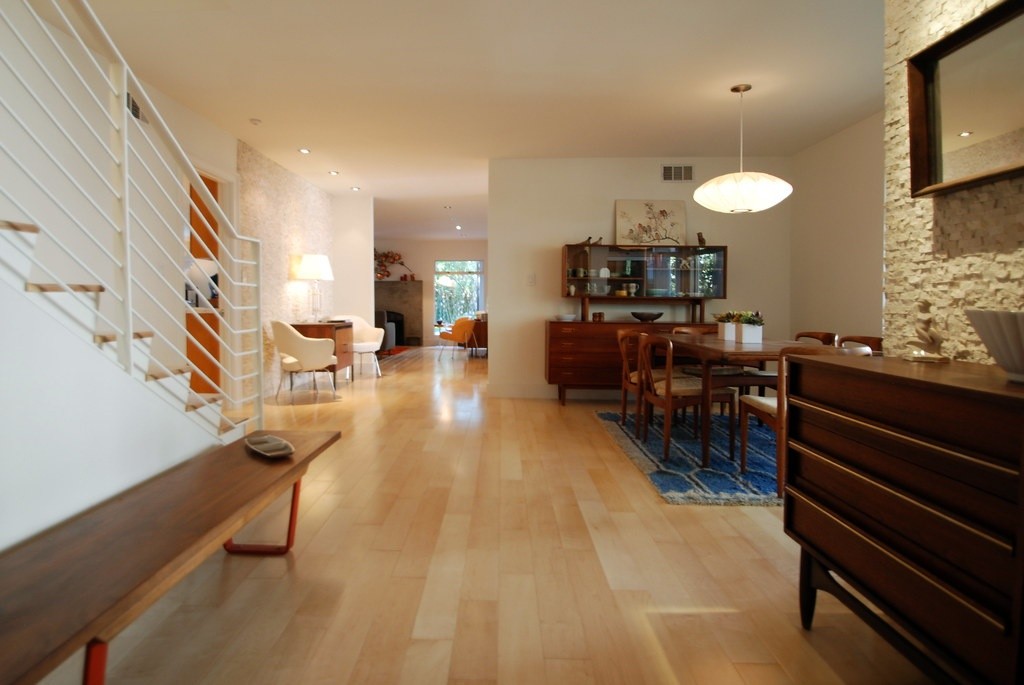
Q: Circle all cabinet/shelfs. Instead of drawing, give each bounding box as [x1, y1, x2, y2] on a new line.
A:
[779, 338, 1024, 685]
[545, 242, 729, 410]
[291, 321, 356, 389]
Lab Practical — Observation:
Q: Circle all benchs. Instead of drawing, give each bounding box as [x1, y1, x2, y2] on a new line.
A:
[0, 429, 346, 685]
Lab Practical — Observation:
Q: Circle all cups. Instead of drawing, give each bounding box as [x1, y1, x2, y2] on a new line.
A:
[588, 268, 599, 277]
[576, 267, 587, 277]
[670, 291, 704, 298]
[622, 282, 639, 296]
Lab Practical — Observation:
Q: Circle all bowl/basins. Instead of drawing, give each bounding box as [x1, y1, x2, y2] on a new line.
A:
[615, 290, 628, 296]
[631, 312, 664, 323]
[555, 314, 577, 321]
[647, 289, 667, 297]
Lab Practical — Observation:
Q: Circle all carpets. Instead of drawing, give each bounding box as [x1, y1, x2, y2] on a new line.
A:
[596, 411, 786, 506]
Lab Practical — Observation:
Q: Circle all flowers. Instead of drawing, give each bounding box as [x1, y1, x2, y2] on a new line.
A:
[732, 311, 766, 327]
[712, 309, 735, 322]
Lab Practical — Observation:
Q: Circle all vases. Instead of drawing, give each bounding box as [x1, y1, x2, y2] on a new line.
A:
[732, 323, 763, 344]
[719, 321, 736, 340]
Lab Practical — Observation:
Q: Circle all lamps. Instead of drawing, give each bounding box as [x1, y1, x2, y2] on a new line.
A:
[288, 253, 335, 321]
[691, 83, 793, 214]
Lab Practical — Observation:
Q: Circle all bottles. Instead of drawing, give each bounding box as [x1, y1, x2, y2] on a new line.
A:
[569, 283, 576, 296]
[599, 266, 610, 277]
[593, 312, 604, 323]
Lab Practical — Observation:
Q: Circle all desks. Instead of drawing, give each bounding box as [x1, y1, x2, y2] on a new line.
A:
[457, 320, 488, 354]
[654, 334, 815, 470]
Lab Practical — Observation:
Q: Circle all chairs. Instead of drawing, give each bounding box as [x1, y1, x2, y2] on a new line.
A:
[375, 310, 396, 359]
[270, 319, 338, 401]
[329, 315, 385, 377]
[617, 326, 884, 498]
[437, 319, 478, 361]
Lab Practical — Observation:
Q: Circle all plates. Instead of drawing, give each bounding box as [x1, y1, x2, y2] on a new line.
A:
[245, 435, 295, 457]
